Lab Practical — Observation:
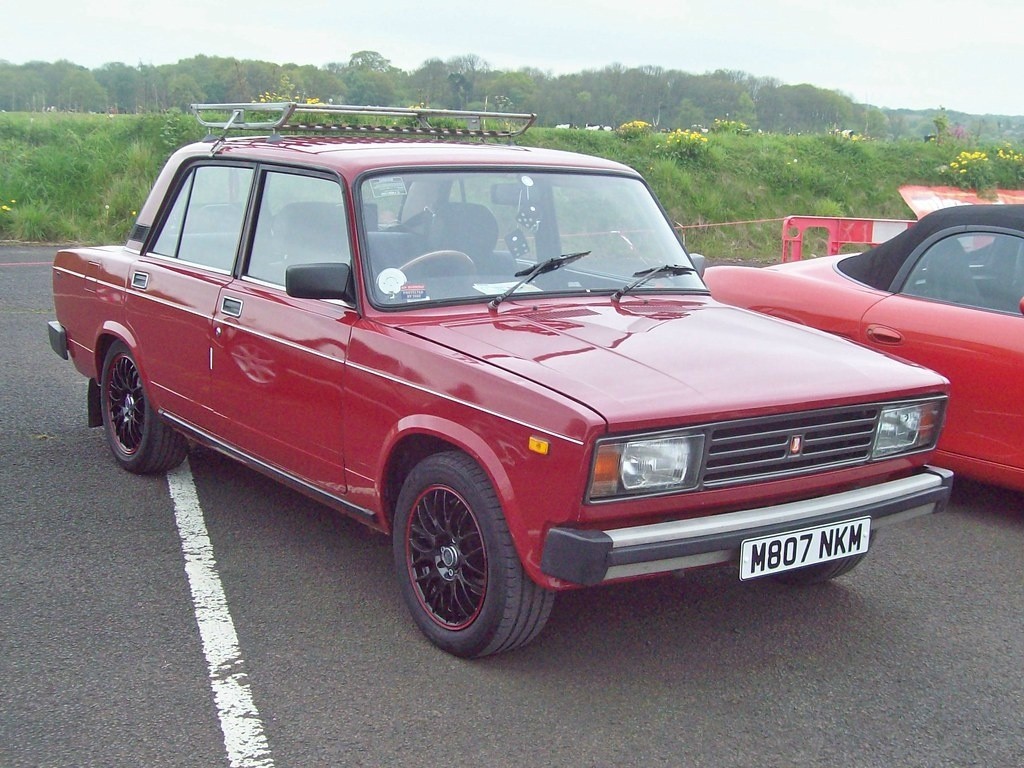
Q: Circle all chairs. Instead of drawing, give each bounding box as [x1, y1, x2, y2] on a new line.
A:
[925, 238, 982, 304]
[986, 236, 1024, 303]
[271, 202, 350, 263]
[423, 203, 503, 276]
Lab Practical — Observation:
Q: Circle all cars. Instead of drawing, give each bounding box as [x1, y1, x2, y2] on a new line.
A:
[702, 201, 1023, 496]
[48, 101, 955, 662]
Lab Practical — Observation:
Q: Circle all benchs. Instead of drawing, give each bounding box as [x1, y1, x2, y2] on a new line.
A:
[183, 204, 419, 267]
